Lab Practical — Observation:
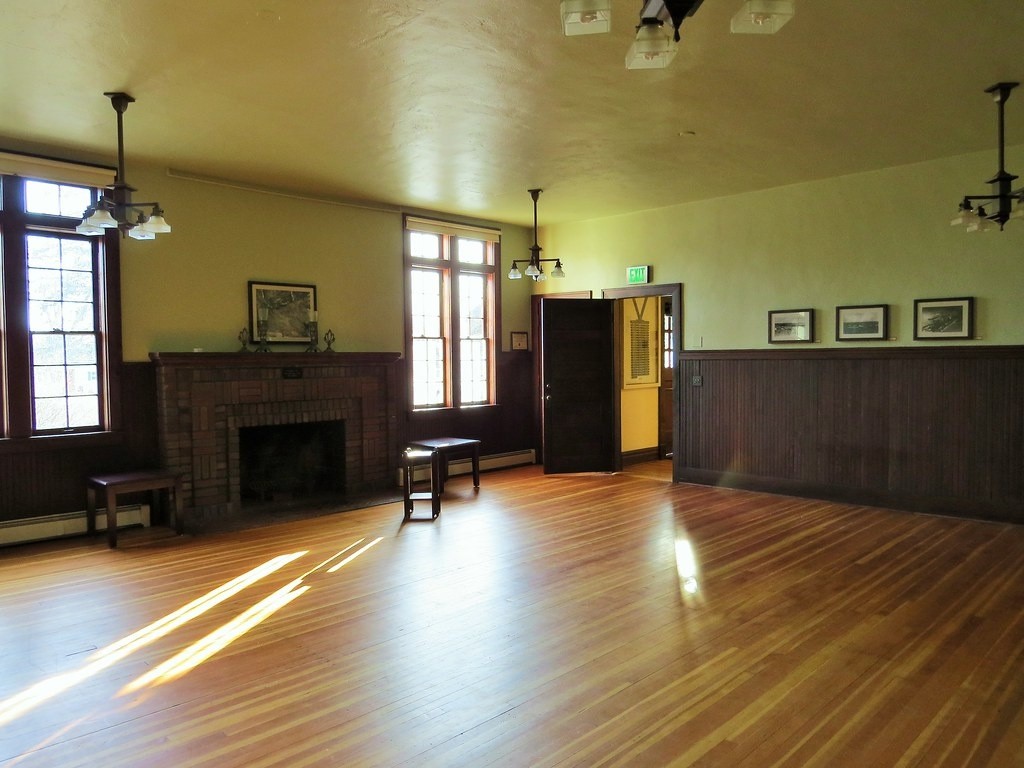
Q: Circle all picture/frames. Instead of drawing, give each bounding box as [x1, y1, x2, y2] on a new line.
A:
[247, 280, 318, 344]
[511, 332, 528, 351]
[767, 308, 814, 344]
[912, 296, 974, 341]
[835, 304, 888, 341]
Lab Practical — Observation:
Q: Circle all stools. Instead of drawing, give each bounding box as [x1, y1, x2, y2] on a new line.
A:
[85, 466, 185, 550]
[409, 436, 481, 493]
[402, 447, 441, 520]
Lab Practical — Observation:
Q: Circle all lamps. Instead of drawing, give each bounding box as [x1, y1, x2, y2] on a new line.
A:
[507, 189, 565, 283]
[76, 92, 172, 240]
[561, 0, 794, 70]
[949, 82, 1024, 232]
